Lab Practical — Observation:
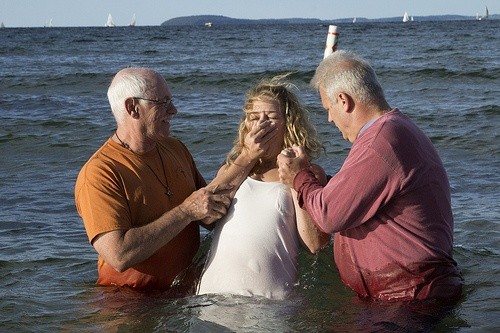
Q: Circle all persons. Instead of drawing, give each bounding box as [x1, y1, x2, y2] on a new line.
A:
[73, 67, 234, 290]
[202, 50, 458, 302]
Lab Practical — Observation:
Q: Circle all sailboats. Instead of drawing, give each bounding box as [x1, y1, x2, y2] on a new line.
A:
[128, 13, 138, 27]
[402, 12, 411, 23]
[105, 12, 116, 28]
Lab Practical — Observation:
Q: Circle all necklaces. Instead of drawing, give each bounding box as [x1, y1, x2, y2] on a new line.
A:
[116, 130, 173, 199]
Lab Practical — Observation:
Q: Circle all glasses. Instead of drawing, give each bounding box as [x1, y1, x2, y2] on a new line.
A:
[134, 96, 174, 109]
